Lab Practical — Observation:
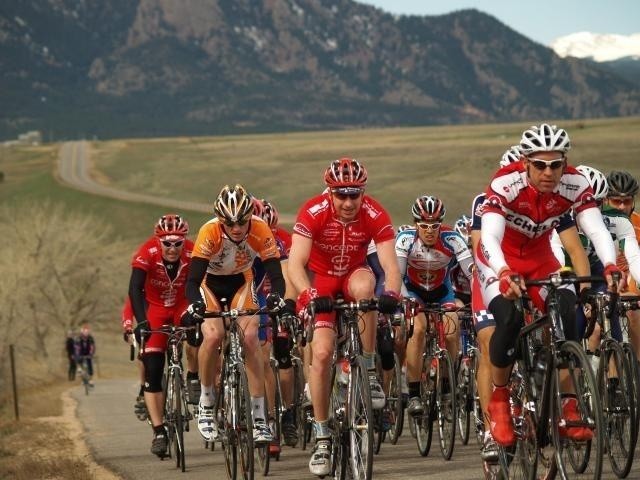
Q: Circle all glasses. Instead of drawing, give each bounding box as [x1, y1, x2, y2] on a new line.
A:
[224, 220, 248, 226]
[160, 239, 184, 247]
[417, 222, 442, 231]
[332, 192, 361, 200]
[526, 158, 565, 169]
[609, 198, 633, 206]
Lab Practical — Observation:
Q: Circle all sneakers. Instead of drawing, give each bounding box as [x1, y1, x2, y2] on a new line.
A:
[252, 418, 275, 442]
[271, 443, 280, 456]
[309, 432, 332, 475]
[282, 411, 298, 446]
[134, 396, 148, 421]
[151, 428, 168, 454]
[368, 371, 386, 410]
[187, 372, 201, 405]
[198, 400, 218, 442]
[407, 397, 424, 414]
[482, 438, 499, 458]
[488, 383, 515, 446]
[560, 397, 593, 440]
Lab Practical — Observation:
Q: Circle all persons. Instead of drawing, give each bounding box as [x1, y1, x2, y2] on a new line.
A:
[121, 120, 640, 480]
[72, 323, 96, 389]
[65, 330, 77, 383]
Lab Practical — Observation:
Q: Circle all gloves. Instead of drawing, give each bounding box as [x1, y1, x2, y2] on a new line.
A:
[603, 264, 627, 283]
[499, 270, 525, 295]
[134, 320, 151, 343]
[123, 330, 134, 345]
[181, 300, 206, 324]
[308, 296, 332, 318]
[378, 291, 403, 315]
[266, 292, 287, 315]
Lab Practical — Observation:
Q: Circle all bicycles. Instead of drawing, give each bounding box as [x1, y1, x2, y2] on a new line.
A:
[126, 328, 192, 470]
[578, 285, 637, 474]
[189, 301, 267, 475]
[264, 313, 291, 461]
[412, 301, 456, 460]
[444, 304, 473, 445]
[299, 299, 379, 475]
[480, 278, 601, 476]
[379, 304, 406, 442]
[74, 353, 97, 398]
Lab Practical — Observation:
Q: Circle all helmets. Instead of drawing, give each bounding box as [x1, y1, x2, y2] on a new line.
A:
[323, 159, 367, 194]
[251, 196, 268, 224]
[456, 216, 473, 245]
[519, 124, 570, 156]
[575, 165, 609, 200]
[500, 146, 523, 168]
[262, 199, 278, 226]
[214, 185, 254, 223]
[606, 170, 639, 198]
[412, 195, 446, 223]
[155, 215, 189, 235]
